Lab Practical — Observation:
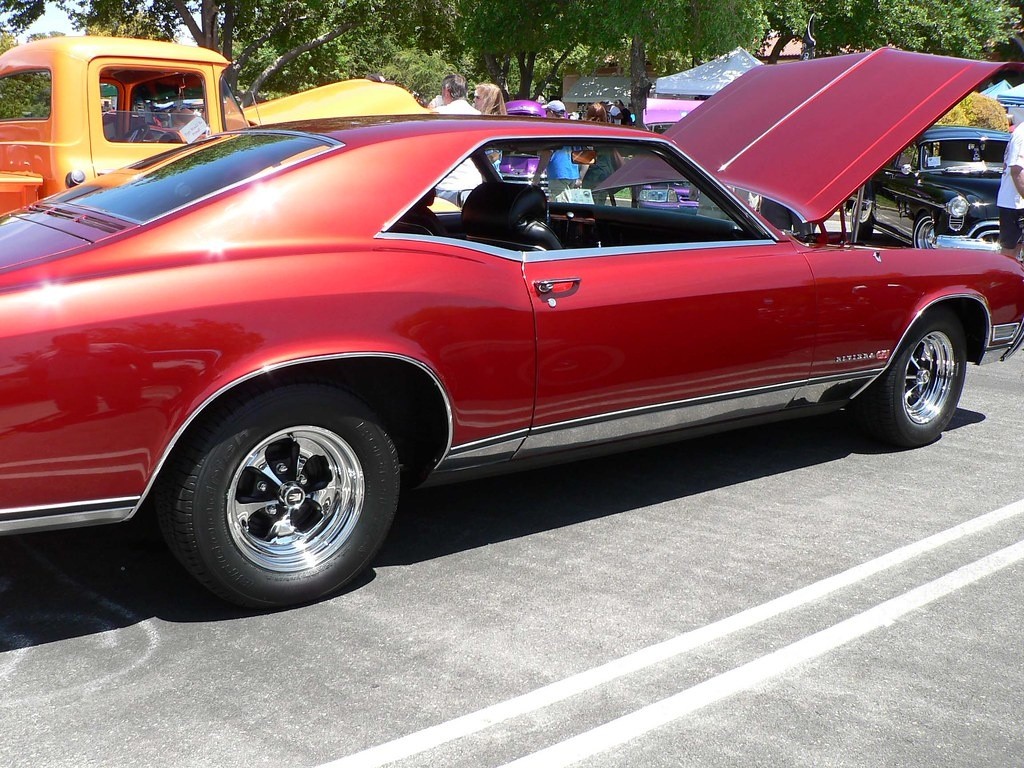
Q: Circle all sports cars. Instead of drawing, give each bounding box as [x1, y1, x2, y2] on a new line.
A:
[1, 45, 1024, 617]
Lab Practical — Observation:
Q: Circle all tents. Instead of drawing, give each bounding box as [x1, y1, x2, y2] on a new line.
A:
[642, 46, 765, 125]
[981, 80, 1024, 114]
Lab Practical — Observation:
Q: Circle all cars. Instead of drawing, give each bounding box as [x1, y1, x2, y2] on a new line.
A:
[0, 31, 470, 220]
[631, 100, 709, 210]
[865, 125, 1024, 248]
[494, 95, 569, 197]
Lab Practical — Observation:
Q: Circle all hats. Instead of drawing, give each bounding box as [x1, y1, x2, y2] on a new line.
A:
[602, 100, 613, 106]
[541, 100, 565, 114]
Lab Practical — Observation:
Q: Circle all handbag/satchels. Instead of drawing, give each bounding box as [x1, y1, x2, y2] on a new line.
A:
[556, 183, 595, 203]
[609, 148, 629, 195]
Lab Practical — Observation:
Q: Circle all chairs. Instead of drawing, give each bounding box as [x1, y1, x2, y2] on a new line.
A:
[462, 182, 564, 252]
[401, 187, 449, 238]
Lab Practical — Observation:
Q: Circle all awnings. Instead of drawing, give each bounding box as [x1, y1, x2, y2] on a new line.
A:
[562, 77, 632, 104]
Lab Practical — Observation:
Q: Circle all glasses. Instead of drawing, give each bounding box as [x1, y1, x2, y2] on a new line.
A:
[546, 110, 548, 114]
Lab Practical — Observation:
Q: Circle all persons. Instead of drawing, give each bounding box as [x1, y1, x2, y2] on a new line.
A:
[733, 187, 816, 237]
[999, 120, 1024, 259]
[101, 72, 636, 207]
